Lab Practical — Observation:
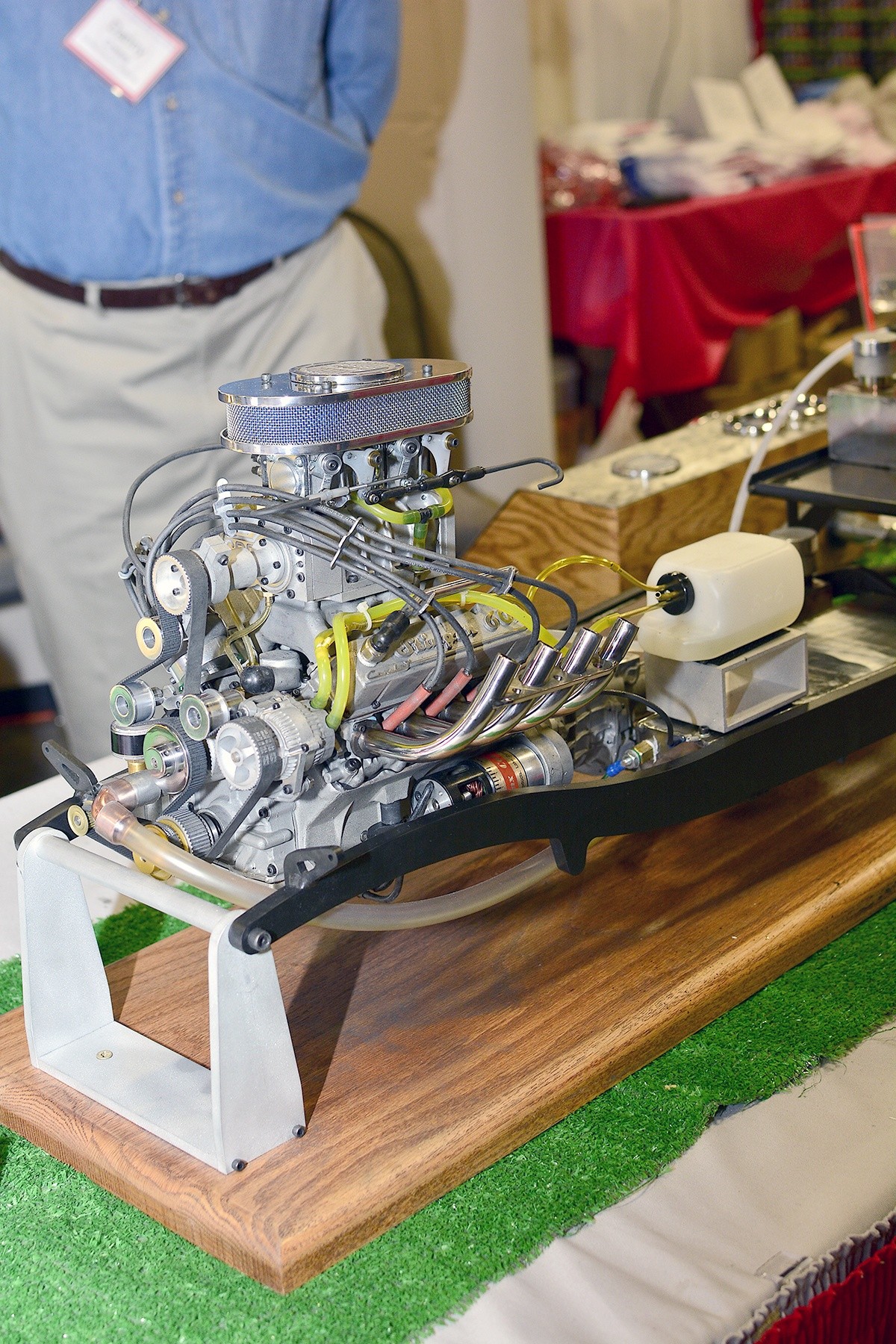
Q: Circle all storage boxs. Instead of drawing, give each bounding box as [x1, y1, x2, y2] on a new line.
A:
[669, 307, 861, 425]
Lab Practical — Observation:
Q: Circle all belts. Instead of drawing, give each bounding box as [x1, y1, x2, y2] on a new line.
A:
[0, 245, 306, 309]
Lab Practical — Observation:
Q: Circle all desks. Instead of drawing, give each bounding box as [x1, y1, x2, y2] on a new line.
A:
[545, 156, 895, 433]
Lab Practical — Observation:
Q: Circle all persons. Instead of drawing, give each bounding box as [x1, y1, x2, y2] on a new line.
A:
[1, 0, 391, 756]
